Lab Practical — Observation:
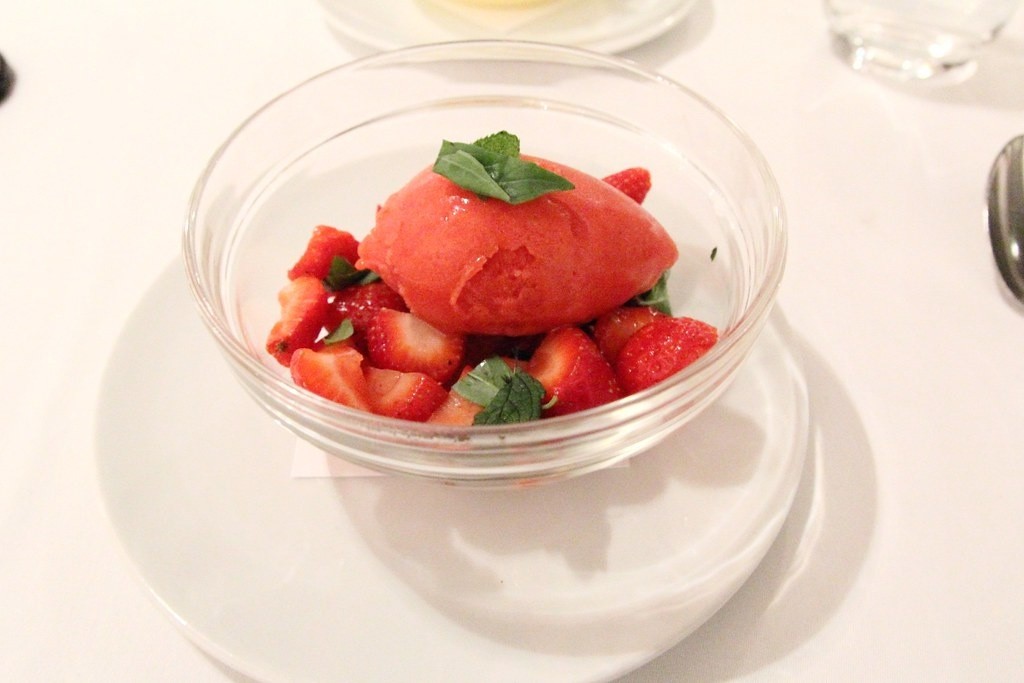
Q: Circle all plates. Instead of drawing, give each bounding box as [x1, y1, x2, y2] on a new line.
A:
[91, 250, 809, 683]
[315, 0, 700, 56]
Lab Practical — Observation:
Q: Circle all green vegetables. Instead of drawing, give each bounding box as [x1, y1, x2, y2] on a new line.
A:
[323, 131, 672, 427]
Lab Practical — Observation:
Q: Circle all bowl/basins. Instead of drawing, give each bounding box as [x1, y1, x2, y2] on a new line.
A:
[182, 39, 788, 489]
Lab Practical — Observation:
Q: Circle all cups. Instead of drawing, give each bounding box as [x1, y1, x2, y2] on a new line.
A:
[827, 0, 1018, 91]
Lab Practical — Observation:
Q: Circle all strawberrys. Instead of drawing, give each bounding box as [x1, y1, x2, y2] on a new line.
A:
[266, 168, 720, 431]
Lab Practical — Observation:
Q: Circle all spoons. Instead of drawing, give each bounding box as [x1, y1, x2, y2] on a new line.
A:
[986, 134, 1024, 302]
